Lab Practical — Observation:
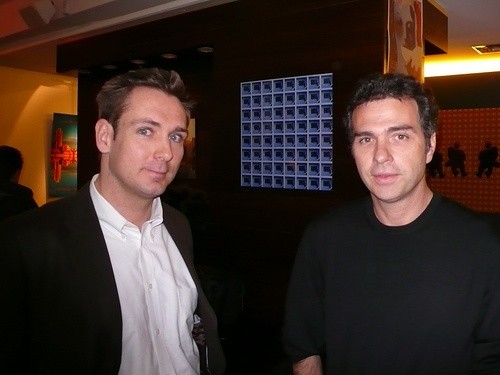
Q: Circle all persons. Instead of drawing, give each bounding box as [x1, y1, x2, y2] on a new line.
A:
[279, 72, 500, 375]
[0, 66, 226, 375]
[0, 145, 39, 217]
